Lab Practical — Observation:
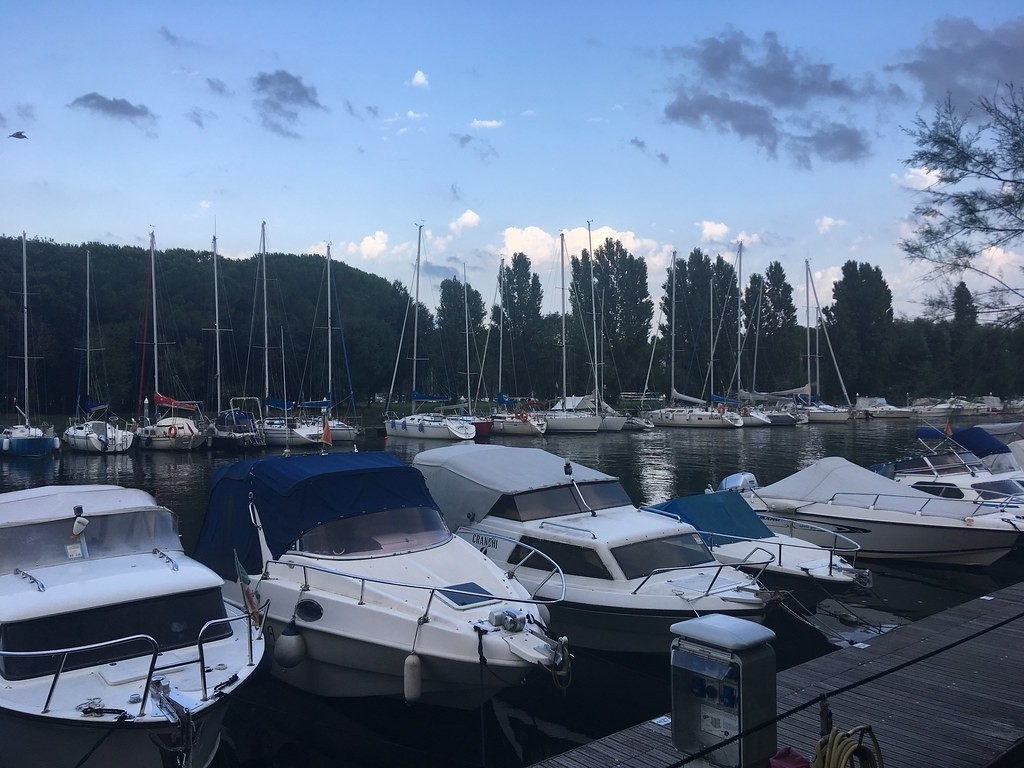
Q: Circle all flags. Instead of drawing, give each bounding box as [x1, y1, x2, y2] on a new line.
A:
[322, 416, 333, 446]
[944, 420, 953, 436]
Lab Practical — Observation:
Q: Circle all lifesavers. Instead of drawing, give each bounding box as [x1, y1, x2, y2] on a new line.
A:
[167, 426, 177, 437]
[718, 405, 725, 413]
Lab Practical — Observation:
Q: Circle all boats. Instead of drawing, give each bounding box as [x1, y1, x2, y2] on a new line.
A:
[634, 484, 875, 598]
[186, 447, 573, 713]
[862, 417, 1024, 513]
[721, 456, 1024, 569]
[0, 483, 270, 768]
[409, 441, 774, 657]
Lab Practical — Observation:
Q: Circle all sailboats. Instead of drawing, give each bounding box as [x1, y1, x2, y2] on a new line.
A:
[198, 235, 266, 451]
[382, 222, 477, 442]
[0, 231, 61, 459]
[129, 224, 210, 451]
[244, 220, 364, 448]
[438, 220, 1024, 436]
[62, 249, 135, 454]
